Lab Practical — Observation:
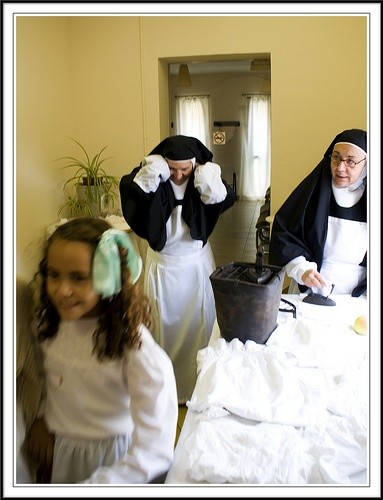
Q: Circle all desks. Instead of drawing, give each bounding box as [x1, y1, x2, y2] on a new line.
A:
[163, 292, 369, 484]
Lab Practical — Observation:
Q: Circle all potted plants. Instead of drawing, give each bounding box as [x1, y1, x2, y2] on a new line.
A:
[54, 139, 120, 219]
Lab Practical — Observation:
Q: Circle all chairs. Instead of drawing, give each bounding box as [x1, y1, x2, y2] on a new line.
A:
[255, 185, 270, 254]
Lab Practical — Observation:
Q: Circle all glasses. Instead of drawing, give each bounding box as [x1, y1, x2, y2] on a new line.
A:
[329, 154, 366, 168]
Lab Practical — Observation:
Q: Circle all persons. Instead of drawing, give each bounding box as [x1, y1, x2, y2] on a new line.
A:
[118, 134, 237, 402]
[267, 129, 368, 298]
[27, 217, 177, 485]
